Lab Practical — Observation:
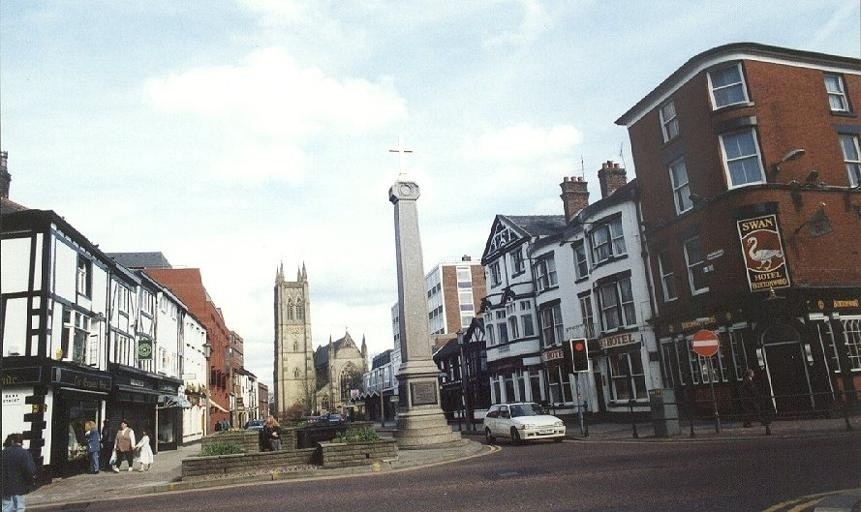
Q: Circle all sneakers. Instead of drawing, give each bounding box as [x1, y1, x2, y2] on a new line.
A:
[114, 467, 151, 472]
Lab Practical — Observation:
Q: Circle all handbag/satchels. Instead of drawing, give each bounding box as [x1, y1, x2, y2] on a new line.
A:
[109, 449, 117, 464]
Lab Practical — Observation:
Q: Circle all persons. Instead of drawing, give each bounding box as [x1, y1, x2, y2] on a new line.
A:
[263, 415, 281, 451]
[0, 433, 40, 512]
[80, 418, 154, 474]
[215, 418, 229, 432]
[738, 367, 764, 427]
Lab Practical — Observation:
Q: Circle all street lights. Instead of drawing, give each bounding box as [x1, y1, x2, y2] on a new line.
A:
[454, 328, 472, 433]
[378, 365, 386, 426]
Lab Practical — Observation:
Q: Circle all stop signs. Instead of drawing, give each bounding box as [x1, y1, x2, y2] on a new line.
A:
[693, 329, 721, 358]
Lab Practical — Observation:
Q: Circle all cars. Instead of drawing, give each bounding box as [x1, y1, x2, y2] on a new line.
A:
[308, 413, 346, 425]
[483, 401, 568, 444]
[245, 419, 264, 432]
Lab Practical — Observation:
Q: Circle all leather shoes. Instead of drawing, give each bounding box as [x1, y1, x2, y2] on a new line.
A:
[88, 470, 99, 474]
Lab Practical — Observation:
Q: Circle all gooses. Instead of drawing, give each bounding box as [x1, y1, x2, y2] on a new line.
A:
[746, 235, 783, 272]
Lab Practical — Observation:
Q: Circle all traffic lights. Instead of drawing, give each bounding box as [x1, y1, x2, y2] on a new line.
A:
[571, 337, 590, 373]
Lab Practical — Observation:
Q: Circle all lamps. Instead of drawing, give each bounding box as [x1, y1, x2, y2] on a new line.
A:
[773, 149, 807, 167]
